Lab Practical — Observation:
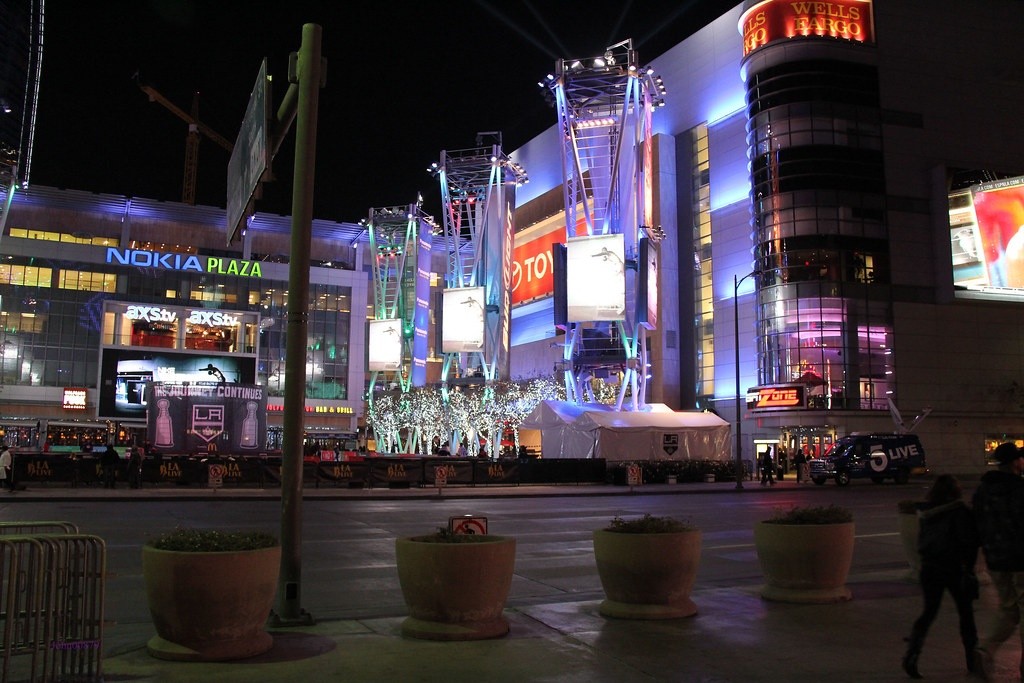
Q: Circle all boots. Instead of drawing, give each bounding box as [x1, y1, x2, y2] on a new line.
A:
[957, 611, 983, 672]
[902, 617, 930, 679]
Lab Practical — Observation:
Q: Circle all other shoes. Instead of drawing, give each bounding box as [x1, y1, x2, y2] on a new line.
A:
[974, 649, 994, 682]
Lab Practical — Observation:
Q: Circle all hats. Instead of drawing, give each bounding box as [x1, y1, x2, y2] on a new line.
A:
[992, 443, 1024, 466]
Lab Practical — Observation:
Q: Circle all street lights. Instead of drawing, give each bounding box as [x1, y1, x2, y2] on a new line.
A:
[735, 269, 763, 488]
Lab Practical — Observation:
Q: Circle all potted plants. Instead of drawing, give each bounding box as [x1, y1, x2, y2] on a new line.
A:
[753, 506, 862, 604]
[143, 529, 283, 661]
[593, 514, 705, 621]
[395, 527, 516, 641]
[899, 500, 925, 577]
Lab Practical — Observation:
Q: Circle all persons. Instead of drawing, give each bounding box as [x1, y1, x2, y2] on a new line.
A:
[974, 442, 1024, 683]
[127, 446, 144, 489]
[806, 448, 816, 463]
[458, 443, 466, 457]
[0, 445, 15, 493]
[100, 444, 121, 489]
[477, 448, 488, 459]
[901, 474, 980, 679]
[304, 442, 321, 454]
[437, 444, 450, 456]
[794, 449, 806, 483]
[760, 447, 777, 484]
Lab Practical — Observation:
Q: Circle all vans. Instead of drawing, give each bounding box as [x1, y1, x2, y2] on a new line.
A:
[808, 431, 926, 488]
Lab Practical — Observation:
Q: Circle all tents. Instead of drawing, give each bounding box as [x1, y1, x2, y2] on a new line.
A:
[519, 400, 732, 468]
